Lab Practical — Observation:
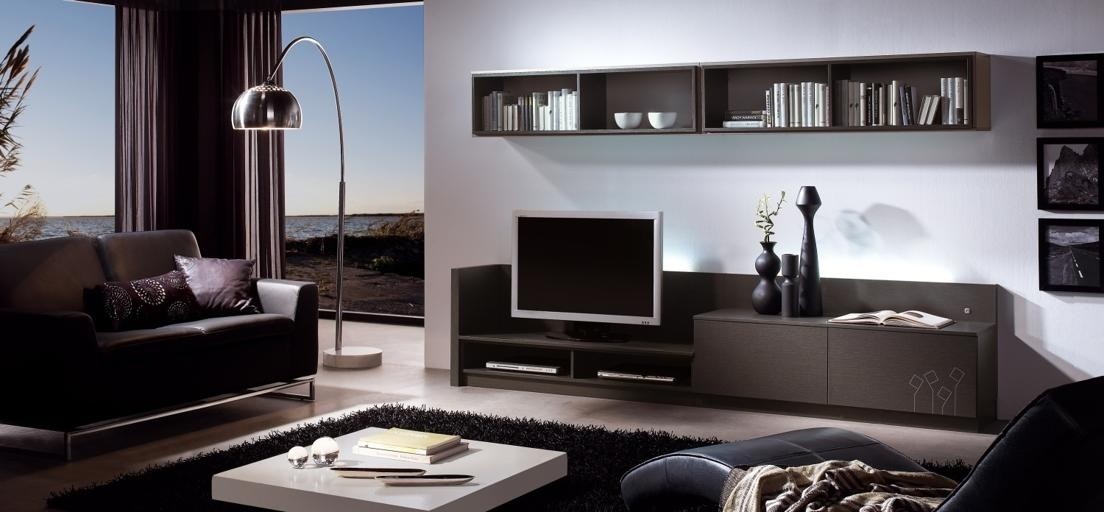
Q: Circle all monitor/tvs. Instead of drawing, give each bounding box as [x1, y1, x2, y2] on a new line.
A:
[512, 209, 663, 343]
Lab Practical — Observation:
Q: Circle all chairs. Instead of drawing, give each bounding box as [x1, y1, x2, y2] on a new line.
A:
[618, 374, 1104, 512]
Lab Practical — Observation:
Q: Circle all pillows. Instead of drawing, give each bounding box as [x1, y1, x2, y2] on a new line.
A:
[93, 229, 202, 283]
[171, 253, 261, 314]
[1, 235, 108, 313]
[94, 270, 205, 332]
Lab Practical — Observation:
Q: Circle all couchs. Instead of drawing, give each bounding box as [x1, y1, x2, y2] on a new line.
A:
[1, 227, 318, 463]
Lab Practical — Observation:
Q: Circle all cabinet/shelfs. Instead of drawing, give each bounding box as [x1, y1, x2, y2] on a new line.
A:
[693, 306, 998, 434]
[701, 49, 991, 133]
[449, 265, 694, 407]
[471, 65, 697, 135]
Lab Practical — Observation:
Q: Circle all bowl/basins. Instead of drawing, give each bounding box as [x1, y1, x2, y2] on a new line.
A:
[614, 112, 677, 128]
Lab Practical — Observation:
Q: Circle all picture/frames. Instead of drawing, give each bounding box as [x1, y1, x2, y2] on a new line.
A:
[1038, 219, 1104, 293]
[1035, 55, 1104, 128]
[1036, 137, 1104, 211]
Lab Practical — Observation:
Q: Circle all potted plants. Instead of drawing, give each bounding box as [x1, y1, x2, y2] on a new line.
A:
[752, 185, 787, 316]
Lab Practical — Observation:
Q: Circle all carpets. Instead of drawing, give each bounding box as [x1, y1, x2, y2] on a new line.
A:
[38, 404, 974, 512]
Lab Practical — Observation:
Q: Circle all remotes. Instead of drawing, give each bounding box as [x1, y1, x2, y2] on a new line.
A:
[330, 468, 425, 478]
[375, 475, 473, 486]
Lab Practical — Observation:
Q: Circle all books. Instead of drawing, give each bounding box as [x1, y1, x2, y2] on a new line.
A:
[828, 307, 955, 330]
[481, 88, 577, 132]
[354, 425, 468, 466]
[723, 79, 829, 128]
[835, 74, 969, 127]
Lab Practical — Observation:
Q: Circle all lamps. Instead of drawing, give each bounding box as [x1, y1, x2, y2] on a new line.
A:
[228, 34, 383, 371]
